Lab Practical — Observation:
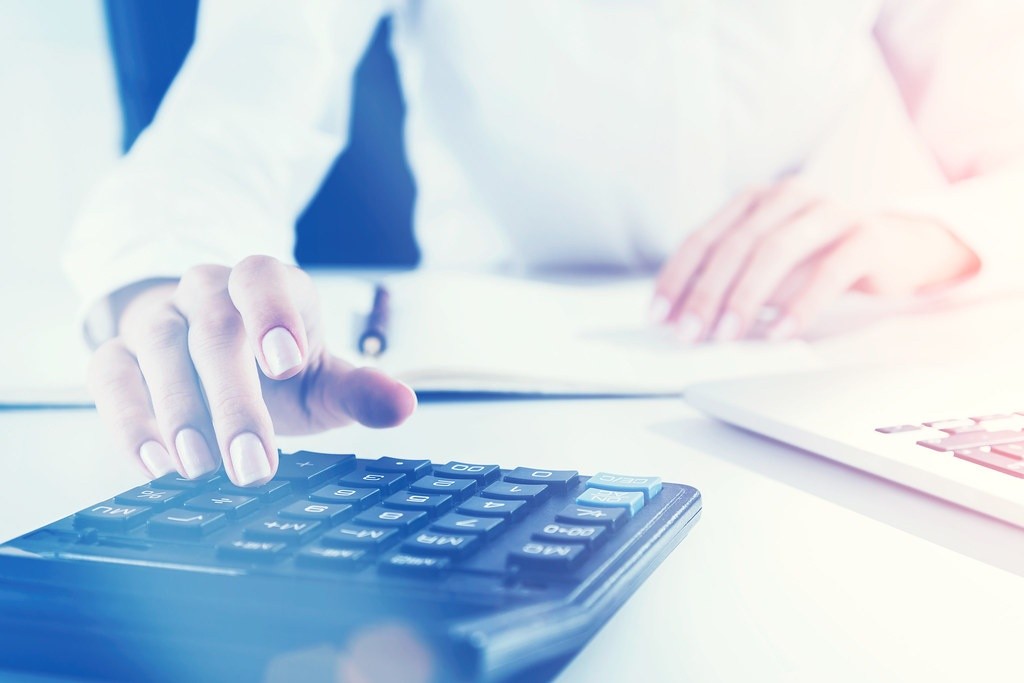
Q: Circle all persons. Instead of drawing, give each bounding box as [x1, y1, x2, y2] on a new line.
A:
[75, 0, 1024, 487]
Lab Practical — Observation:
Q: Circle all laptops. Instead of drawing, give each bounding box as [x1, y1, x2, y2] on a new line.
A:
[687, 361, 1024, 527]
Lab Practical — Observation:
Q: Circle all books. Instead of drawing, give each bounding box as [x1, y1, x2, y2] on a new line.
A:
[0, 272, 735, 410]
